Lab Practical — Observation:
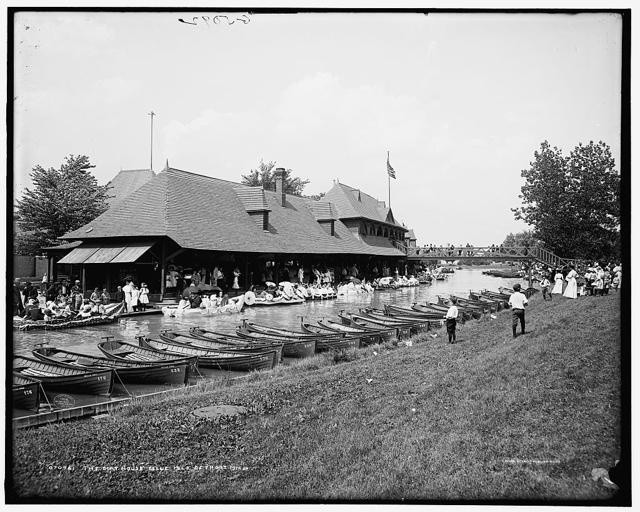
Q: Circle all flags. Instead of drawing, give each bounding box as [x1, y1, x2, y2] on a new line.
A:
[386, 151, 396, 181]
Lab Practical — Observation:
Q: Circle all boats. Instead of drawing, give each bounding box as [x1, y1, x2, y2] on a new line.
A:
[481, 260, 523, 280]
[374, 264, 454, 291]
[12, 354, 115, 398]
[11, 372, 41, 410]
[135, 286, 541, 370]
[98, 336, 198, 383]
[13, 299, 126, 332]
[32, 346, 188, 386]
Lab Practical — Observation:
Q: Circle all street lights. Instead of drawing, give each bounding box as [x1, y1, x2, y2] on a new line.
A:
[148, 111, 156, 170]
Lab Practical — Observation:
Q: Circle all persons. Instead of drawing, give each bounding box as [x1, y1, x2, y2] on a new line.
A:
[491, 244, 504, 257]
[522, 241, 529, 256]
[539, 260, 621, 299]
[508, 283, 529, 339]
[443, 296, 459, 343]
[535, 240, 544, 257]
[13, 265, 432, 320]
[416, 243, 474, 257]
